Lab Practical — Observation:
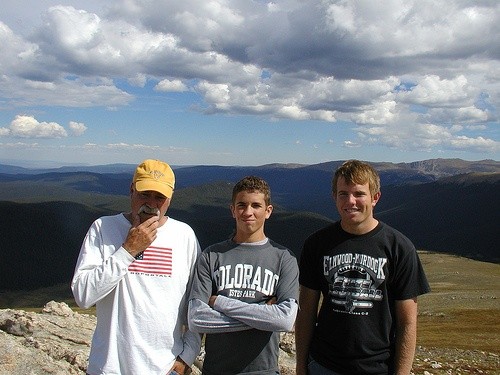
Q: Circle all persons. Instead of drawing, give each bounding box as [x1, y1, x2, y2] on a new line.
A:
[188, 176, 299, 375]
[294, 161, 430, 375]
[71, 160, 204, 375]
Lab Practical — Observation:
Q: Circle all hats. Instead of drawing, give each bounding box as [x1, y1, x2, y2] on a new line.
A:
[133, 159, 175, 199]
